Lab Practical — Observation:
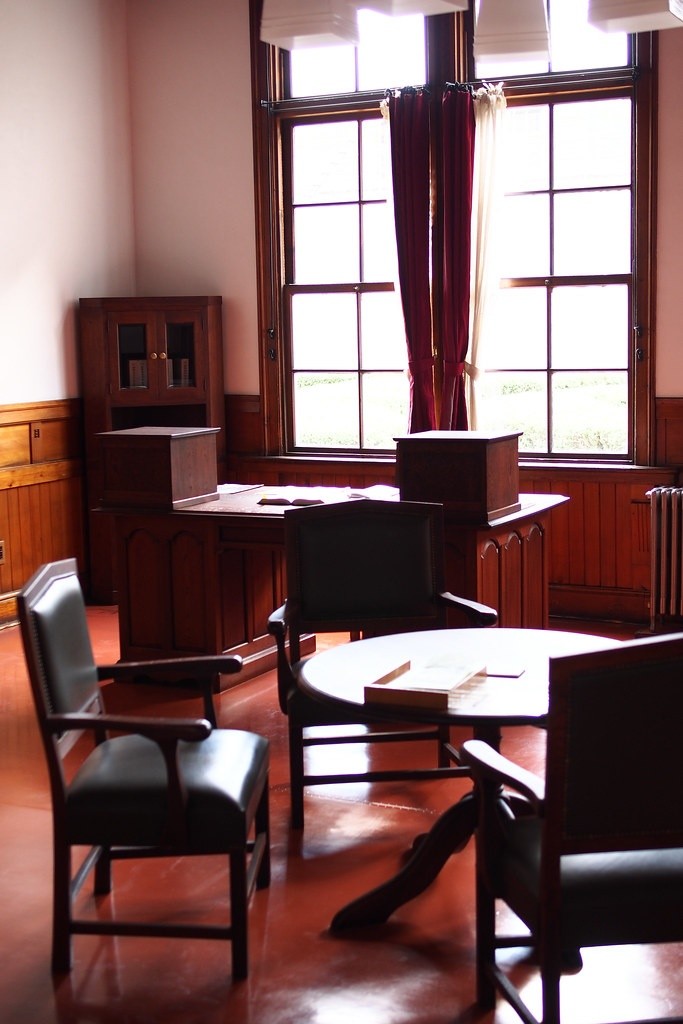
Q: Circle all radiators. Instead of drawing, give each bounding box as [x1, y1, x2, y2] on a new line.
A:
[635, 486, 683, 637]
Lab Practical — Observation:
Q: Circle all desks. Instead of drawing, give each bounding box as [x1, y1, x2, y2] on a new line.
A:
[91, 426, 569, 693]
[299, 629, 628, 976]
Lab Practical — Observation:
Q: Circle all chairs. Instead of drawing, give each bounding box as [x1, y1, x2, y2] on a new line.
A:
[268, 499, 473, 825]
[16, 554, 273, 986]
[462, 633, 683, 1024]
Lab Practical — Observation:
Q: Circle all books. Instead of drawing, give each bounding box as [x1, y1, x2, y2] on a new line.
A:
[217, 478, 263, 495]
[128, 357, 190, 386]
[257, 485, 399, 506]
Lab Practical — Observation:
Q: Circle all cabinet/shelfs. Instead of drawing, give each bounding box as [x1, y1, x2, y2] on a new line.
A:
[77, 295, 226, 607]
